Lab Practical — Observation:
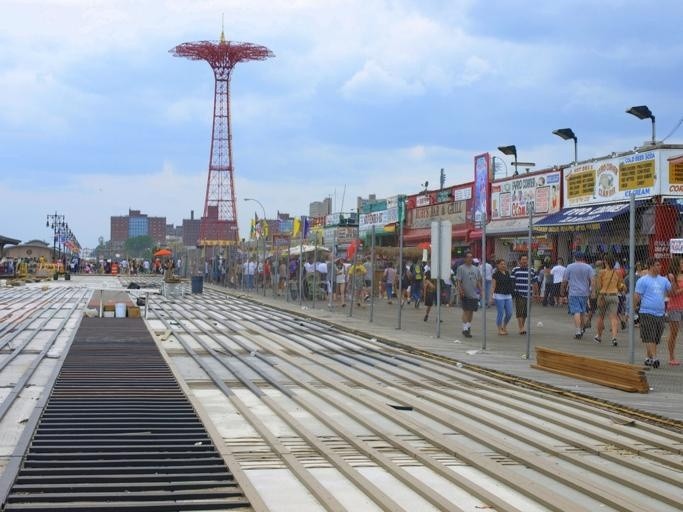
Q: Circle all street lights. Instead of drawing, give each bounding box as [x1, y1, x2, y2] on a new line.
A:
[44, 211, 66, 261]
[242, 198, 266, 222]
[51, 219, 83, 260]
[549, 125, 581, 162]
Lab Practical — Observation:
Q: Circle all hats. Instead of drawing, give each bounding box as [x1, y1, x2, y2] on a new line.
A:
[573, 251, 585, 258]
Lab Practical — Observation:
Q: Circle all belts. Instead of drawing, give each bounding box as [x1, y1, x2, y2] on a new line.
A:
[598, 292, 617, 296]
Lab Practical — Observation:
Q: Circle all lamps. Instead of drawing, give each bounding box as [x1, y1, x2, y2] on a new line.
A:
[551, 127, 578, 163]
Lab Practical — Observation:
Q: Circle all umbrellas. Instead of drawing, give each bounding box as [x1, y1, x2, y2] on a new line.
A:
[282, 244, 331, 255]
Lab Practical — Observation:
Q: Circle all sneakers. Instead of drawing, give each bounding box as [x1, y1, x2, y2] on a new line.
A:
[519, 328, 526, 335]
[592, 335, 601, 344]
[497, 328, 508, 336]
[574, 330, 584, 340]
[611, 337, 617, 347]
[538, 296, 570, 310]
[329, 294, 444, 324]
[643, 358, 652, 366]
[667, 359, 680, 366]
[652, 359, 659, 369]
[462, 327, 472, 338]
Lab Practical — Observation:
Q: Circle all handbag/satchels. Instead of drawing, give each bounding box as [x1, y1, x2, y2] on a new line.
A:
[596, 294, 606, 309]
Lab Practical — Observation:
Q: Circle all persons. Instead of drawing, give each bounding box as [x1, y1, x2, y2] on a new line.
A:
[383, 256, 446, 323]
[0, 255, 65, 276]
[455, 253, 538, 338]
[535, 251, 682, 369]
[120, 257, 181, 274]
[67, 256, 110, 273]
[196, 252, 286, 291]
[279, 254, 371, 308]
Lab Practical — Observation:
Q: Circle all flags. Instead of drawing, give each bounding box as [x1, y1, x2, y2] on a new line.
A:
[250, 209, 324, 241]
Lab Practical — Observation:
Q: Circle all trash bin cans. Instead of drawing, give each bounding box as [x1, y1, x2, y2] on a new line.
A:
[191, 276, 202, 293]
